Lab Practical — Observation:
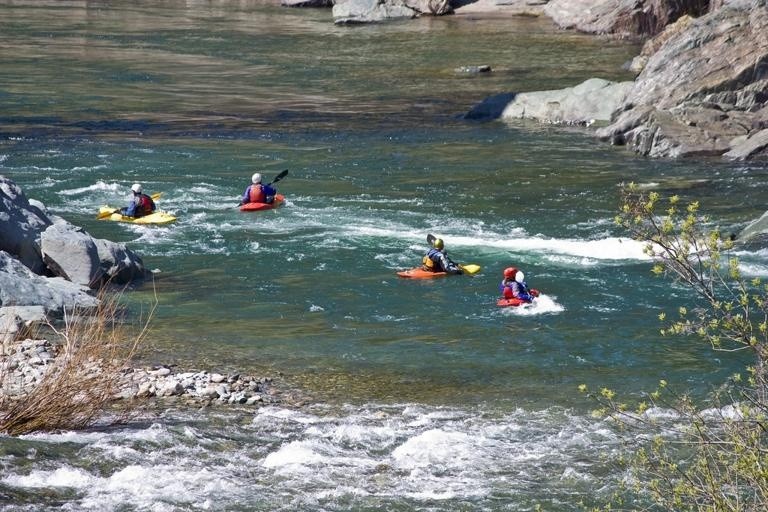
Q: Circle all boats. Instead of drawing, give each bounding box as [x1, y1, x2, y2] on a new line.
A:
[239, 192, 284, 212]
[394, 263, 481, 280]
[99, 204, 177, 226]
[493, 288, 541, 308]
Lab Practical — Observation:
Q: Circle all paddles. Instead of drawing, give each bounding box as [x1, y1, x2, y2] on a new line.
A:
[97, 192, 162, 220]
[426, 234, 474, 278]
[514, 271, 534, 301]
[238, 170, 289, 207]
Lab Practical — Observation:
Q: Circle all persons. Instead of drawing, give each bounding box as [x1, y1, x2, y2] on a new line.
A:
[116, 182, 156, 220]
[497, 266, 535, 303]
[421, 238, 460, 275]
[240, 172, 275, 205]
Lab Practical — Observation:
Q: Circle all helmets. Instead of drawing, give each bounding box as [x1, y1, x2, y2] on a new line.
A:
[504, 267, 518, 278]
[131, 183, 143, 193]
[432, 238, 444, 249]
[252, 172, 262, 183]
